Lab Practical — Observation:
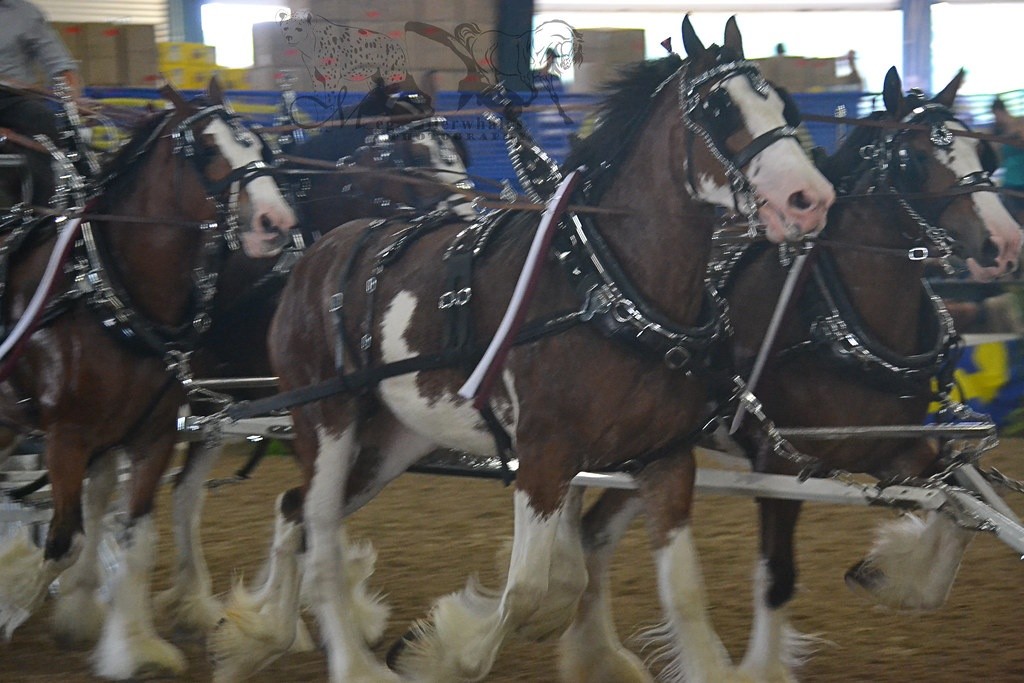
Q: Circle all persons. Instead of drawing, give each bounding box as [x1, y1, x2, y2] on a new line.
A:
[0, 0, 81, 97]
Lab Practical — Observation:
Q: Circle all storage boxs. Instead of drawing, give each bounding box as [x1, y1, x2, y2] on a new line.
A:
[748, 56, 861, 97]
[573, 28, 644, 91]
[251, 0, 498, 92]
[41, 22, 156, 88]
[159, 43, 250, 91]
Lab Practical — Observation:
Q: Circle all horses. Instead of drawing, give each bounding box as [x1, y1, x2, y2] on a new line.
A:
[1, 14, 1024, 683]
